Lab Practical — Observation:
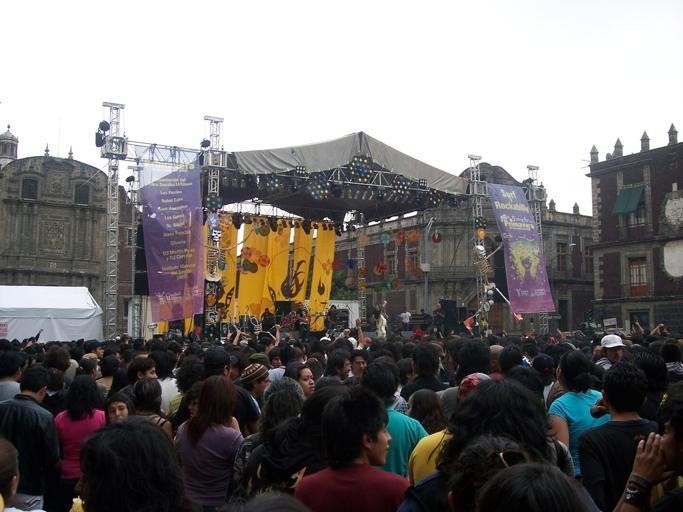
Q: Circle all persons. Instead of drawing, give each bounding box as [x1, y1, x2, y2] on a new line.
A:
[1, 299, 683, 511]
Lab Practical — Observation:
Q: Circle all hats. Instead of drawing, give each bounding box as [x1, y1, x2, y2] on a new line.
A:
[601, 335, 627, 348]
[204, 349, 231, 368]
[240, 364, 269, 384]
